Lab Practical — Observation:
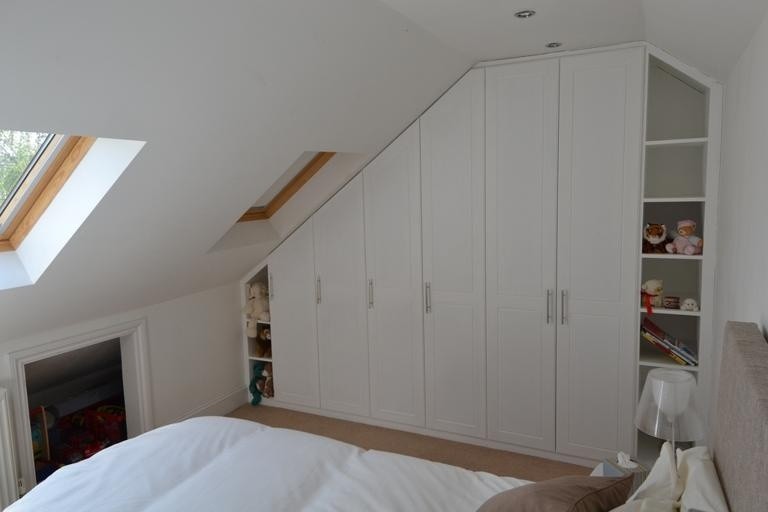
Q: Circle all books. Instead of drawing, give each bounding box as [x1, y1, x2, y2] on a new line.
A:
[640, 317, 699, 367]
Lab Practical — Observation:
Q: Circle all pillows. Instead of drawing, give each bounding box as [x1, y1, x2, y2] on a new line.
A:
[468, 445, 721, 512]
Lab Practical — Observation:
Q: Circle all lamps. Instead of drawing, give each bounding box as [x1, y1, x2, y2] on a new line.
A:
[634, 366, 707, 488]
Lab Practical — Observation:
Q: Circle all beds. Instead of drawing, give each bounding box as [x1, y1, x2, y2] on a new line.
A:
[2, 413, 734, 510]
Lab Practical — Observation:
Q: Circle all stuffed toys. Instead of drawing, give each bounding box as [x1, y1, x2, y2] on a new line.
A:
[249, 364, 264, 405]
[641, 278, 665, 315]
[643, 222, 673, 254]
[681, 297, 700, 313]
[665, 218, 703, 255]
[256, 323, 272, 359]
[241, 280, 270, 338]
[256, 362, 274, 398]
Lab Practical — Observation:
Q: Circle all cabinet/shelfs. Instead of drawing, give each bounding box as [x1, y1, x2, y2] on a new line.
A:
[240, 41, 723, 472]
